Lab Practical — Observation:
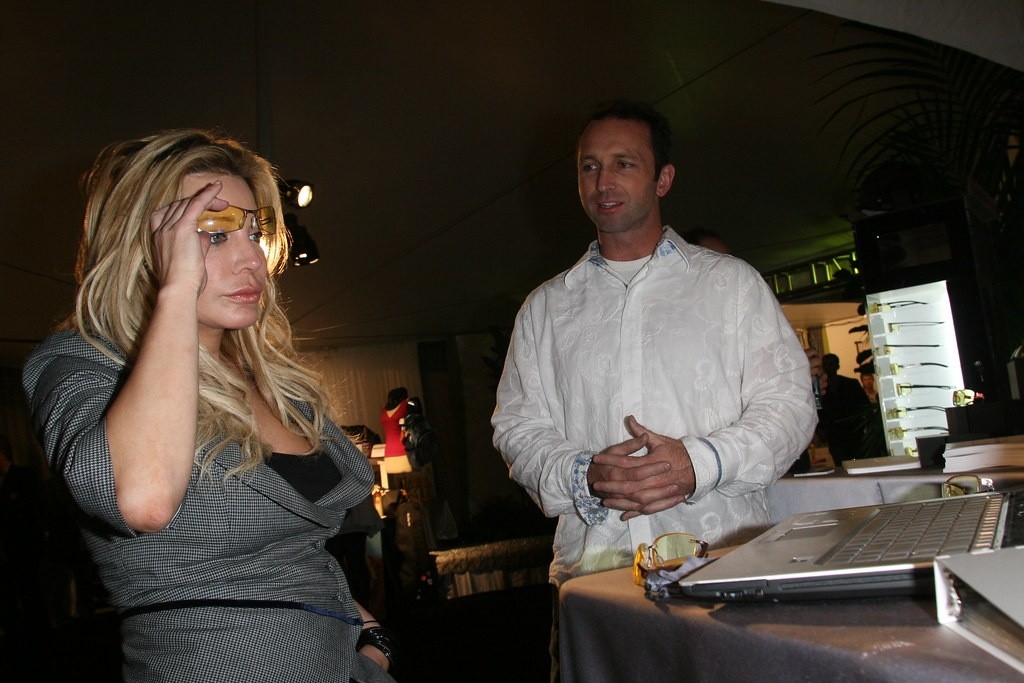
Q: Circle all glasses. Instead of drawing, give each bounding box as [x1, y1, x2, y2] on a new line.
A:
[888, 322, 944, 333]
[953, 388, 975, 408]
[884, 344, 940, 355]
[886, 406, 945, 420]
[197, 205, 277, 235]
[869, 300, 928, 313]
[634, 534, 709, 587]
[889, 363, 948, 376]
[889, 426, 949, 440]
[941, 474, 995, 498]
[905, 447, 917, 455]
[897, 382, 951, 397]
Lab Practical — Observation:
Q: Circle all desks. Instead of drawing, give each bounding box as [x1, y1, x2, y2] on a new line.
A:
[768, 464, 953, 529]
[558, 544, 1024, 683]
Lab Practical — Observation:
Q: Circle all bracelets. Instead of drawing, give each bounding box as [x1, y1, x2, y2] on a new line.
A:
[355, 627, 393, 663]
[364, 620, 378, 623]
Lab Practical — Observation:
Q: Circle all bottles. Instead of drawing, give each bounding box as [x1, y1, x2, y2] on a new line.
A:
[814, 373, 820, 395]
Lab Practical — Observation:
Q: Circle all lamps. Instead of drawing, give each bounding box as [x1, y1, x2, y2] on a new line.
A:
[279, 179, 319, 266]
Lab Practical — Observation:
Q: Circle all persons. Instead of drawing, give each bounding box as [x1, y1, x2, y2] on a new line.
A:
[489, 108, 821, 683]
[815, 353, 888, 468]
[380, 387, 423, 491]
[17, 133, 394, 683]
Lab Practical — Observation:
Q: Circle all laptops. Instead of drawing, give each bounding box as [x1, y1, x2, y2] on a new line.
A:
[667, 476, 1024, 604]
